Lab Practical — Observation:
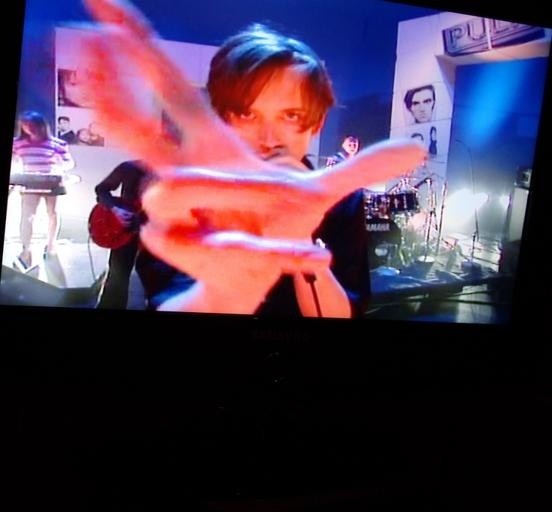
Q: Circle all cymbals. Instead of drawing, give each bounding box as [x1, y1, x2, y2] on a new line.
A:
[316, 156, 343, 162]
[400, 175, 421, 180]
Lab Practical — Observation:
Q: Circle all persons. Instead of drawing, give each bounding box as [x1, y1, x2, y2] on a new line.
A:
[411, 133, 424, 141]
[76, 128, 91, 145]
[95, 162, 174, 310]
[58, 116, 77, 143]
[404, 85, 436, 124]
[71, 0, 427, 319]
[89, 123, 105, 146]
[428, 126, 439, 156]
[324, 134, 359, 168]
[12, 113, 70, 266]
[60, 69, 104, 110]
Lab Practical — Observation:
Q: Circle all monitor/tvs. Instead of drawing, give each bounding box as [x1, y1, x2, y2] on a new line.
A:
[0, 0, 552, 383]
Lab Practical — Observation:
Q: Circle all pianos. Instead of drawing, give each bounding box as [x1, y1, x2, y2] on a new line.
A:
[10, 173, 81, 189]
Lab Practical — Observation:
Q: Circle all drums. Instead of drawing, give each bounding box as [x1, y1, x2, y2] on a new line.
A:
[390, 191, 420, 213]
[362, 192, 391, 218]
[364, 219, 401, 269]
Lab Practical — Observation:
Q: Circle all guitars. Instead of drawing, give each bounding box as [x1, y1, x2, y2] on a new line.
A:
[88, 197, 148, 248]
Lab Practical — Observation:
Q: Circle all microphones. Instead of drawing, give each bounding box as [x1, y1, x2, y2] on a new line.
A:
[262, 145, 317, 284]
